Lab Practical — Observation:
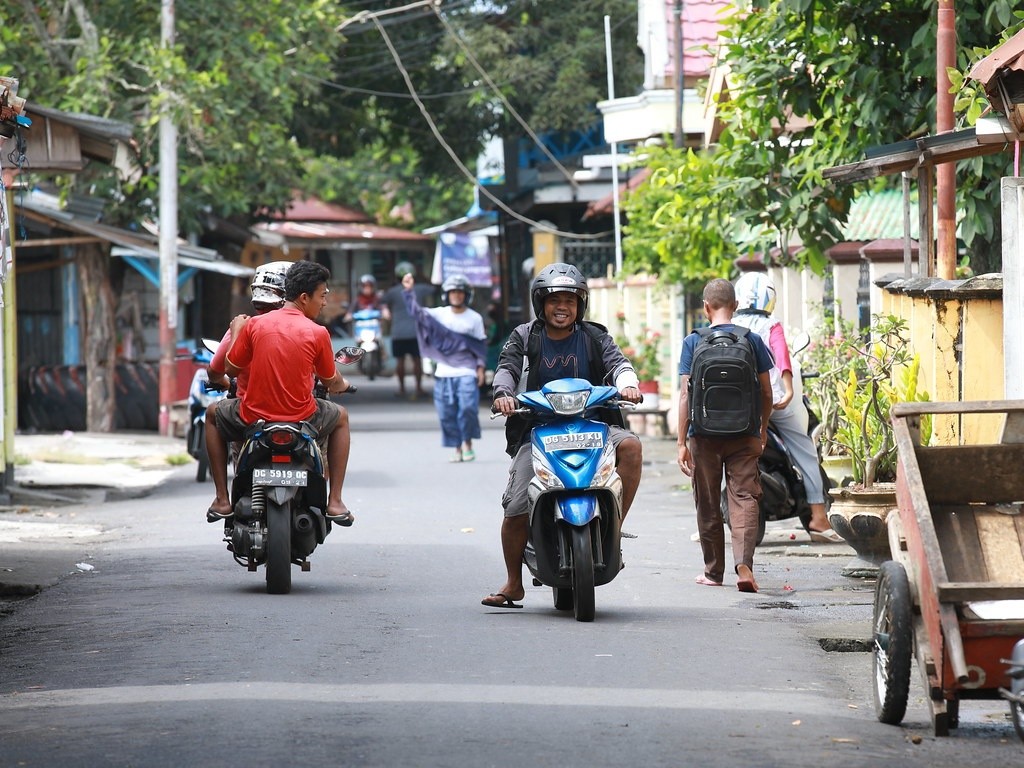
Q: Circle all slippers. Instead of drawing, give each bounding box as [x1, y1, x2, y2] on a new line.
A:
[696, 574, 723, 586]
[808, 528, 846, 543]
[206, 497, 234, 521]
[326, 511, 355, 527]
[481, 594, 523, 609]
[736, 579, 758, 593]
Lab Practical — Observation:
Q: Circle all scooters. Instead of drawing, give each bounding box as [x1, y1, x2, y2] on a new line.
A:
[489, 377, 644, 622]
[186, 336, 367, 595]
[341, 300, 384, 381]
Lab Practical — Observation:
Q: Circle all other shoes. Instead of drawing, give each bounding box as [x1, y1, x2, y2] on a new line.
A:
[462, 449, 476, 463]
[449, 454, 462, 462]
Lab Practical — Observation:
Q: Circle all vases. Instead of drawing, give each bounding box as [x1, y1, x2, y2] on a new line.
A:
[633, 383, 659, 412]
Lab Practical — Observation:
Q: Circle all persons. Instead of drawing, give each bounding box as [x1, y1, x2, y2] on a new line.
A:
[677, 280, 775, 594]
[384, 261, 436, 397]
[206, 258, 354, 527]
[342, 274, 391, 359]
[481, 265, 643, 609]
[401, 272, 487, 462]
[731, 272, 846, 542]
[207, 260, 349, 482]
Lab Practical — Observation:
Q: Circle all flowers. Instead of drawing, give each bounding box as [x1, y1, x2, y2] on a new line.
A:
[619, 313, 667, 374]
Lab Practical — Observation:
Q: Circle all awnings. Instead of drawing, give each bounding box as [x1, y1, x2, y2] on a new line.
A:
[421, 214, 497, 236]
[13, 196, 255, 298]
[247, 217, 434, 254]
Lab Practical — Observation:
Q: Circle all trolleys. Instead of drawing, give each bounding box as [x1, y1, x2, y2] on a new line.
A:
[869, 400, 1024, 736]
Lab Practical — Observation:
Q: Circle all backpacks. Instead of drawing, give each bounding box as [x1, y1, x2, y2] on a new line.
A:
[685, 328, 764, 438]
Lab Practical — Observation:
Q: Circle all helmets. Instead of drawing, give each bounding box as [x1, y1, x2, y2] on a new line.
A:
[395, 261, 416, 278]
[250, 260, 329, 310]
[530, 262, 590, 326]
[361, 274, 375, 285]
[734, 272, 777, 316]
[440, 274, 475, 306]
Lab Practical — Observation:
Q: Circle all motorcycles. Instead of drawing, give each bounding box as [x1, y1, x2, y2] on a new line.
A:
[721, 331, 835, 547]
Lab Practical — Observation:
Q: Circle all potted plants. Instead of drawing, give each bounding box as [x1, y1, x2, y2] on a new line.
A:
[805, 311, 932, 559]
